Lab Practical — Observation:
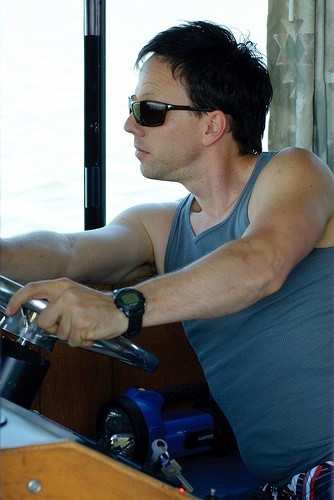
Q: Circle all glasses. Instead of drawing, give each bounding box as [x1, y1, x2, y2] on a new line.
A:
[128, 95, 214, 127]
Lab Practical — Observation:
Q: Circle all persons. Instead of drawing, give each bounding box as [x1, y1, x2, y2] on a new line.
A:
[0, 19, 334, 500]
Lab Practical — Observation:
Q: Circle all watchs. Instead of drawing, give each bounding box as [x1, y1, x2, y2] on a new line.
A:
[113, 287, 147, 336]
